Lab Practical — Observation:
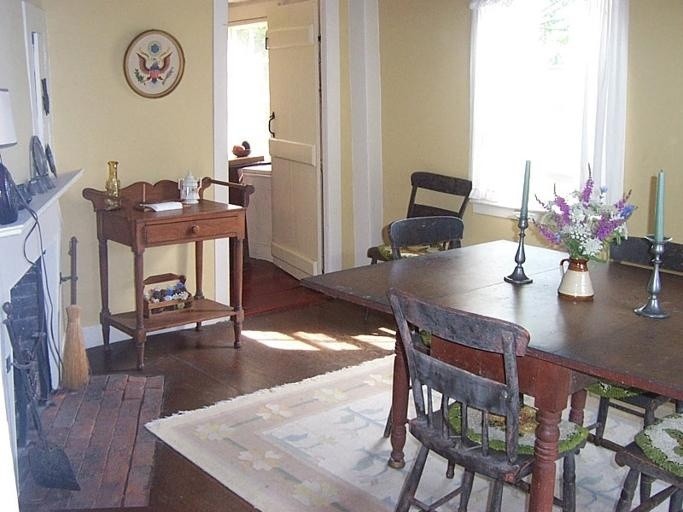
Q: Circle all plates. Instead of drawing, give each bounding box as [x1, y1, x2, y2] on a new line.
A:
[45, 142, 56, 178]
[30, 135, 49, 177]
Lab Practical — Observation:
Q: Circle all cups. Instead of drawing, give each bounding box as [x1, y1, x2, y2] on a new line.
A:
[13, 173, 55, 210]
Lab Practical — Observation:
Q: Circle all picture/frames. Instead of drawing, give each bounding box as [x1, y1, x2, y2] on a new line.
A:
[122, 28, 187, 98]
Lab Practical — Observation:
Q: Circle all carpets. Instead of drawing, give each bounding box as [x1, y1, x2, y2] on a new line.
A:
[143, 351, 683, 512]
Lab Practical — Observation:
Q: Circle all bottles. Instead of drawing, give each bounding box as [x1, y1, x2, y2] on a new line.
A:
[103, 160, 120, 211]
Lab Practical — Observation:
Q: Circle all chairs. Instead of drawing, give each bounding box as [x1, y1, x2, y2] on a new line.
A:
[575, 236, 683, 456]
[363, 170, 472, 322]
[612, 411, 683, 512]
[381, 286, 589, 512]
[378, 213, 466, 439]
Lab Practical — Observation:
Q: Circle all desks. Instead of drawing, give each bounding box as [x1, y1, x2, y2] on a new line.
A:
[300, 241, 683, 469]
[81, 177, 255, 371]
[228, 155, 265, 271]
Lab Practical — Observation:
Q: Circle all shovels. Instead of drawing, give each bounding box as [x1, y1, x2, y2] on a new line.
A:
[2, 302, 81, 492]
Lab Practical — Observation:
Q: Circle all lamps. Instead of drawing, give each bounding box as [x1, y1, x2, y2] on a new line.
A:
[0, 88, 16, 166]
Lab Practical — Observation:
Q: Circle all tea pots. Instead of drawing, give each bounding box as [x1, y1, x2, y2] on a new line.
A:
[176, 170, 203, 205]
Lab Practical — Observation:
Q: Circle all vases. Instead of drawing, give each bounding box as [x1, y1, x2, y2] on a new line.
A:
[557, 256, 595, 302]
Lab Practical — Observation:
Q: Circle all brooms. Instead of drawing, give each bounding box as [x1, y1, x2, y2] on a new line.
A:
[61, 235, 91, 391]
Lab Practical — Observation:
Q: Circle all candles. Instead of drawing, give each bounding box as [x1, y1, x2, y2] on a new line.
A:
[653, 169, 665, 240]
[519, 160, 530, 220]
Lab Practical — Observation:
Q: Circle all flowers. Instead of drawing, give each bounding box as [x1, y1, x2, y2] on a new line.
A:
[146, 282, 189, 310]
[530, 161, 639, 258]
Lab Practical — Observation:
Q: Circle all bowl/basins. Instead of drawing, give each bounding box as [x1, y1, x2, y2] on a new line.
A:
[231, 149, 251, 157]
